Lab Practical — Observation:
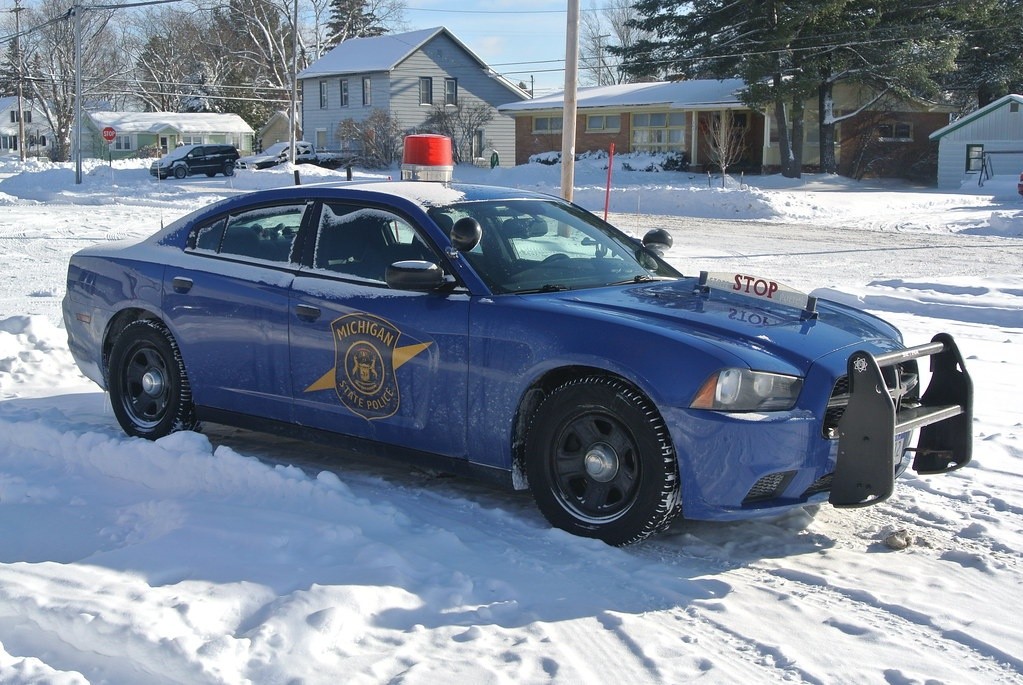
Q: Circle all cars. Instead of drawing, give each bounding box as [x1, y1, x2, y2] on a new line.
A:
[59, 132, 974, 547]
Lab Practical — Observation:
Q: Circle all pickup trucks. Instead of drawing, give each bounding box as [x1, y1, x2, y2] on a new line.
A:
[237, 140, 339, 170]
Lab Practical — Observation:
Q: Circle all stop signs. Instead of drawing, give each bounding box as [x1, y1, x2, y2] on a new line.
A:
[103, 127, 116, 141]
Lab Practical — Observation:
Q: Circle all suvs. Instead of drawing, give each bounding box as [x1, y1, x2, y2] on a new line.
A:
[151, 144, 240, 179]
